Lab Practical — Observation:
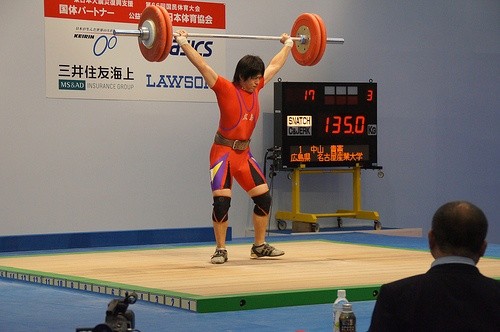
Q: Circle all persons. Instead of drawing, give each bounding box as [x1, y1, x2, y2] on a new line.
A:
[368, 201, 500, 332]
[174, 31, 294, 263]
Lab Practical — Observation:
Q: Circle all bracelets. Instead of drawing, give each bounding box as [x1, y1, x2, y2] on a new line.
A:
[176, 37, 188, 46]
[284, 39, 294, 48]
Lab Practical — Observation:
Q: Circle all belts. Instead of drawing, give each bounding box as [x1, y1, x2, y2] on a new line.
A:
[215, 135, 251, 151]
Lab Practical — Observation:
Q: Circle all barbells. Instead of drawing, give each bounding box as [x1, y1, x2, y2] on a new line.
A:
[114, 6, 345, 66]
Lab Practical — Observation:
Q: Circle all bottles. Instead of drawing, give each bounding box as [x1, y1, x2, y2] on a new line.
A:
[331, 289, 350, 332]
[338, 304, 356, 332]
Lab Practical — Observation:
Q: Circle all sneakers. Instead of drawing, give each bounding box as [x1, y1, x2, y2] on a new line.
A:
[211, 249, 228, 263]
[250, 242, 285, 258]
